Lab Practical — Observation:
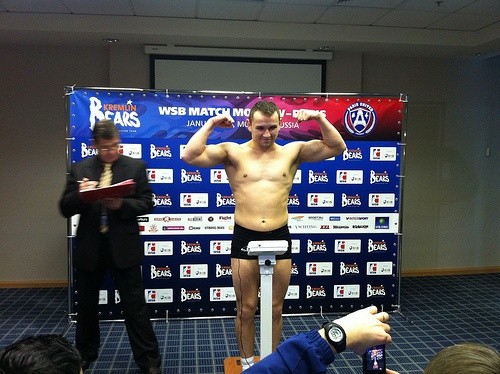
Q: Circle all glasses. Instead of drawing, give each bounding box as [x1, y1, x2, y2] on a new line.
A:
[97, 146, 118, 151]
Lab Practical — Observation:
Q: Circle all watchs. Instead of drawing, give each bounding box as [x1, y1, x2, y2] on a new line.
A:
[322, 320, 346, 354]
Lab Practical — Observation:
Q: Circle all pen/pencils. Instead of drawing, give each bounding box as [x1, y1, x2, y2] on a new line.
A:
[77, 180, 101, 188]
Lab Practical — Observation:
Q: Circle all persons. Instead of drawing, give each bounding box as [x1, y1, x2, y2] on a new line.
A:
[181, 99, 346, 371]
[0, 334, 83, 374]
[239, 305, 500, 374]
[58, 122, 162, 374]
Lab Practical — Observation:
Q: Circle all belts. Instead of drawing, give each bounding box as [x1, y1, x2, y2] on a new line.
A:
[99, 224, 112, 234]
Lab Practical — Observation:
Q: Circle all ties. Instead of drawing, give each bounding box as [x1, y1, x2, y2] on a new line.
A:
[97, 163, 112, 189]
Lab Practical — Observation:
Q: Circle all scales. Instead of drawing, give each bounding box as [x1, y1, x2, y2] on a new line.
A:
[223, 240, 289, 374]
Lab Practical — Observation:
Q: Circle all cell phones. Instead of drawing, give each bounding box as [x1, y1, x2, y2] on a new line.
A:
[362, 344, 386, 374]
